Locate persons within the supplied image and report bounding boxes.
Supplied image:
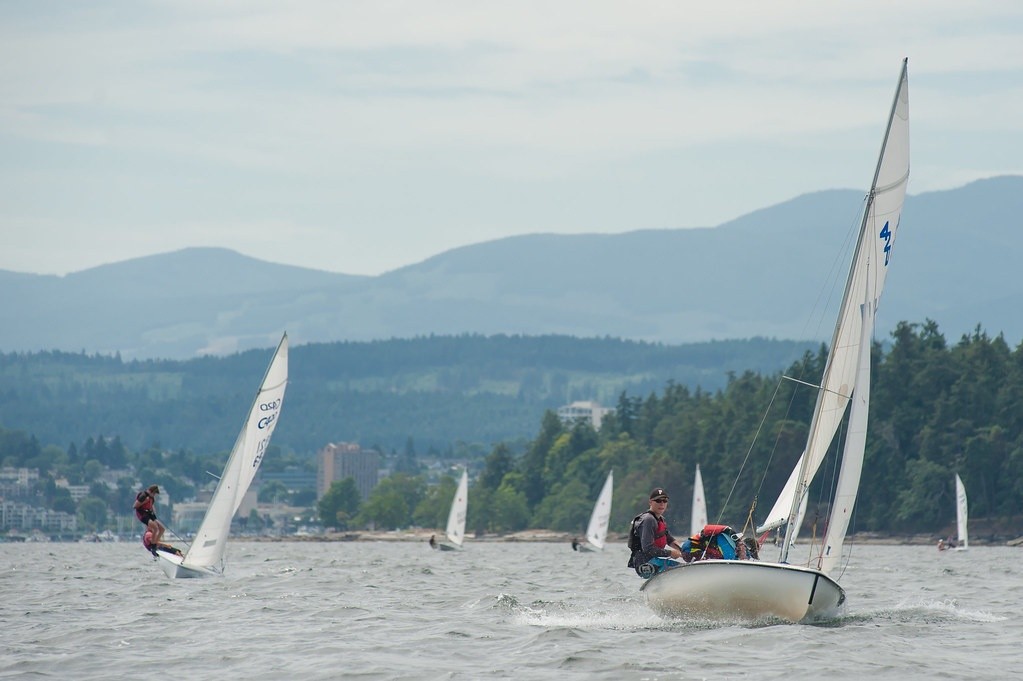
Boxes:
[430,535,434,545]
[132,485,165,558]
[573,539,579,550]
[682,526,760,563]
[143,525,171,552]
[938,539,947,551]
[627,488,682,576]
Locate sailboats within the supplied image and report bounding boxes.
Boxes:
[640,55,913,631]
[946,473,971,553]
[429,465,469,553]
[571,468,615,555]
[154,328,288,581]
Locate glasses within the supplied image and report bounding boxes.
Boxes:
[652,499,668,503]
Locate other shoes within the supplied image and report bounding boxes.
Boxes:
[152,543,159,557]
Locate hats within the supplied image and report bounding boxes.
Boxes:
[650,488,669,500]
[744,538,761,560]
